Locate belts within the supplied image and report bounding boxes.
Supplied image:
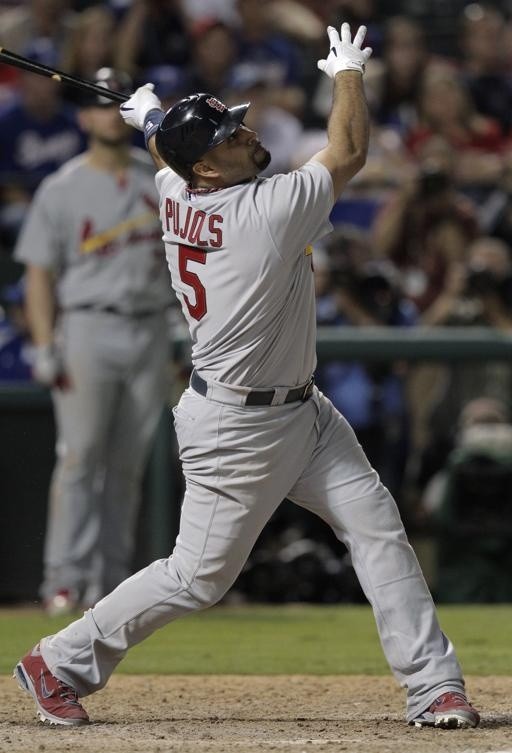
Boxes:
[188,371,317,407]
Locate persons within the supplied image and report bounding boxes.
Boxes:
[12,22,483,729]
[0,0,511,623]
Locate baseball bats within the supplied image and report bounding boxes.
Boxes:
[0,47,129,107]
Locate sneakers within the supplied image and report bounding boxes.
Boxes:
[412,691,480,729]
[11,641,90,726]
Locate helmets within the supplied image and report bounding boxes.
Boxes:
[155,92,251,181]
[73,67,133,107]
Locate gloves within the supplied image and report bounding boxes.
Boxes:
[31,344,63,386]
[316,21,373,79]
[119,81,164,131]
